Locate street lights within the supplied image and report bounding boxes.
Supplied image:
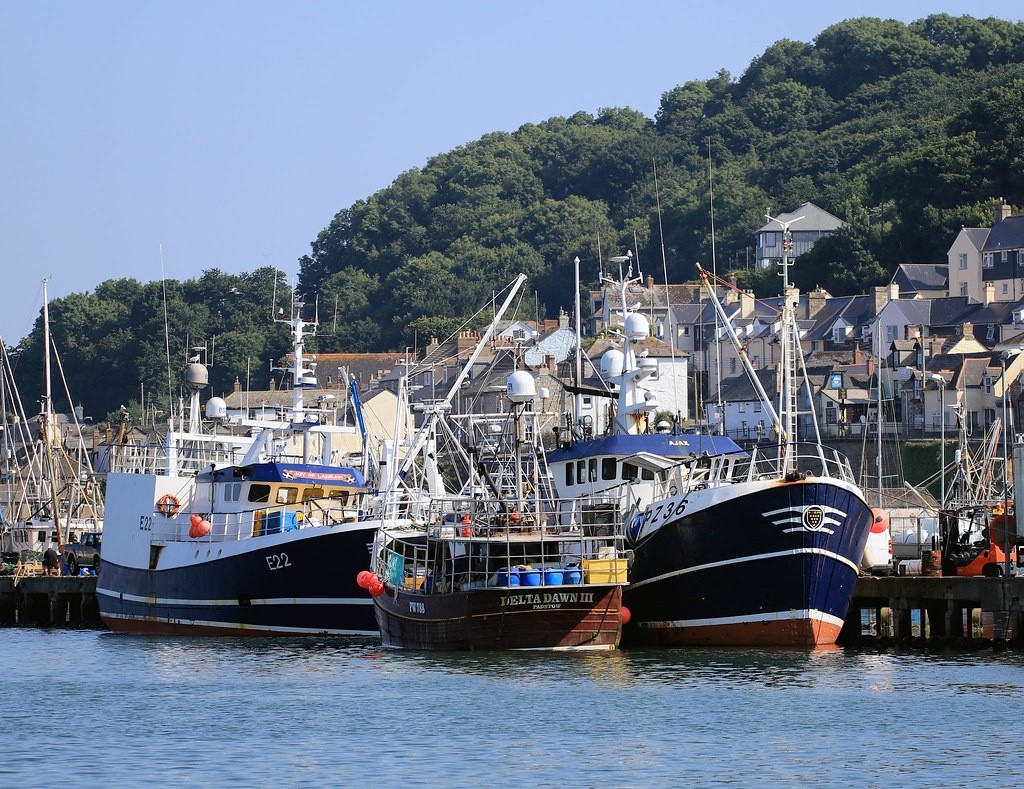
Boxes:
[926,374,946,511]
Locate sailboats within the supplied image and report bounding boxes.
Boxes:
[0,275,106,599]
[846,308,1014,555]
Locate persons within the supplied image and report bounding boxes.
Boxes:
[756,421,762,442]
[838,416,847,438]
[859,414,873,433]
[41,548,58,576]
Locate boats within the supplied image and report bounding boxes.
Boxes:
[368,272,632,647]
[95,244,387,639]
[538,230,754,526]
[610,135,874,648]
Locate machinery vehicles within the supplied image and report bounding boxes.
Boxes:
[939,504,1017,577]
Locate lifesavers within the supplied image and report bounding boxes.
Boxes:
[158,494,180,516]
